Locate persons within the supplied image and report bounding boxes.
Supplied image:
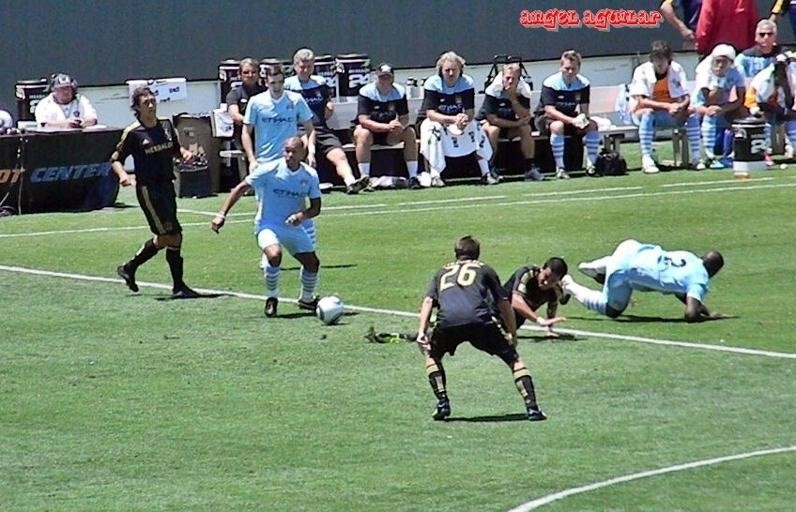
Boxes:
[212,137,321,316]
[35,74,97,128]
[111,87,196,297]
[416,235,545,421]
[557,239,723,321]
[227,0,795,193]
[497,257,569,337]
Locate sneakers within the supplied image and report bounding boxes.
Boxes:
[560,263,605,305]
[173,285,198,297]
[528,409,547,421]
[409,175,445,189]
[347,176,373,194]
[586,164,596,176]
[432,401,450,420]
[525,168,545,181]
[481,166,504,185]
[298,295,319,310]
[642,156,659,173]
[264,297,278,316]
[118,263,138,292]
[688,157,724,170]
[556,166,569,178]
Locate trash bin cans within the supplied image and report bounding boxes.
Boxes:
[731,118,767,161]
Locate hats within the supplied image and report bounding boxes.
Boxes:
[377,63,394,77]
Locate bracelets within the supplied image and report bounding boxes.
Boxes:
[537,317,545,326]
[216,214,228,219]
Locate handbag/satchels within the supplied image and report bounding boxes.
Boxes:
[597,148,627,176]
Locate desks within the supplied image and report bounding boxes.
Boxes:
[0,123,125,217]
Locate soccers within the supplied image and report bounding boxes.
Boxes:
[317,297,344,325]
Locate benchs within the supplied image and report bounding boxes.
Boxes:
[209,76,755,176]
